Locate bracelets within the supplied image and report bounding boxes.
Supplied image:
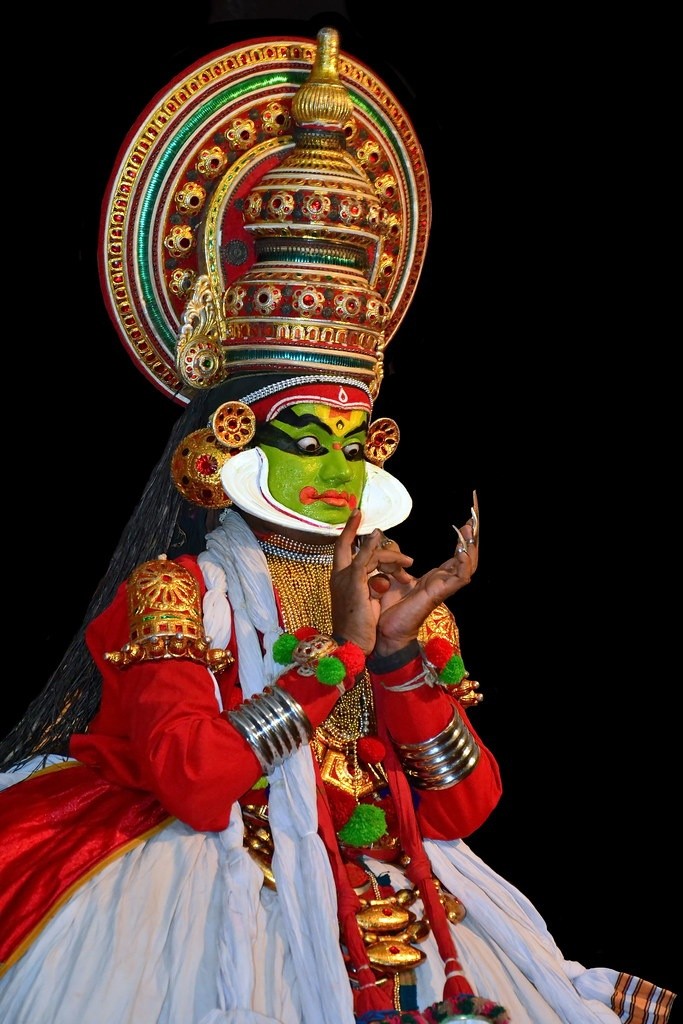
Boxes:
[380,640,484,709]
[272,626,367,694]
[391,704,481,791]
[225,684,312,776]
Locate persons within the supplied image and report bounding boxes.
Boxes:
[0,28,683,1024]
